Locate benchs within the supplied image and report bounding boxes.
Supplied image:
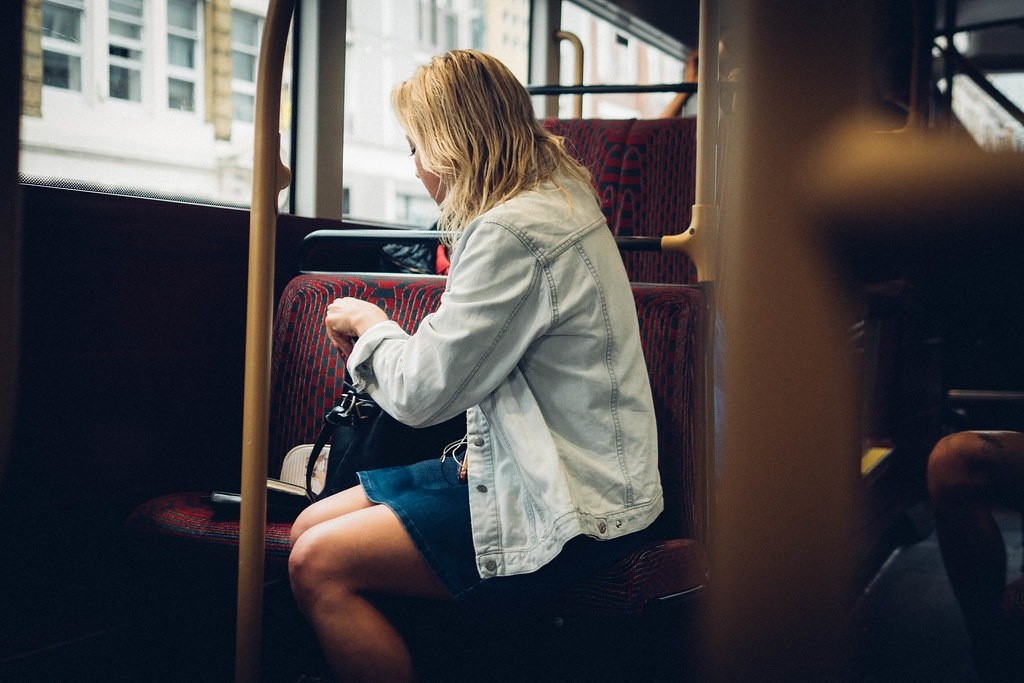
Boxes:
[432,118,700,288]
[127,267,711,626]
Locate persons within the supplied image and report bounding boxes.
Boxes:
[288,49,664,683]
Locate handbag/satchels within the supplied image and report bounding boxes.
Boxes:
[305,366,466,507]
